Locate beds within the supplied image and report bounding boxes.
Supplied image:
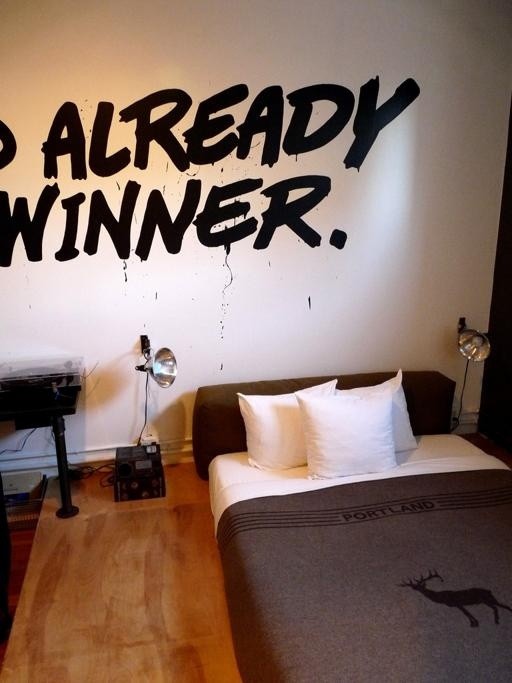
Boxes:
[179,367,512,683]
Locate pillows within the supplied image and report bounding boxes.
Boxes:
[294,387,398,480]
[234,377,339,470]
[335,368,419,453]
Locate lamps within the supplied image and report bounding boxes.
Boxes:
[135,334,178,447]
[449,315,491,434]
